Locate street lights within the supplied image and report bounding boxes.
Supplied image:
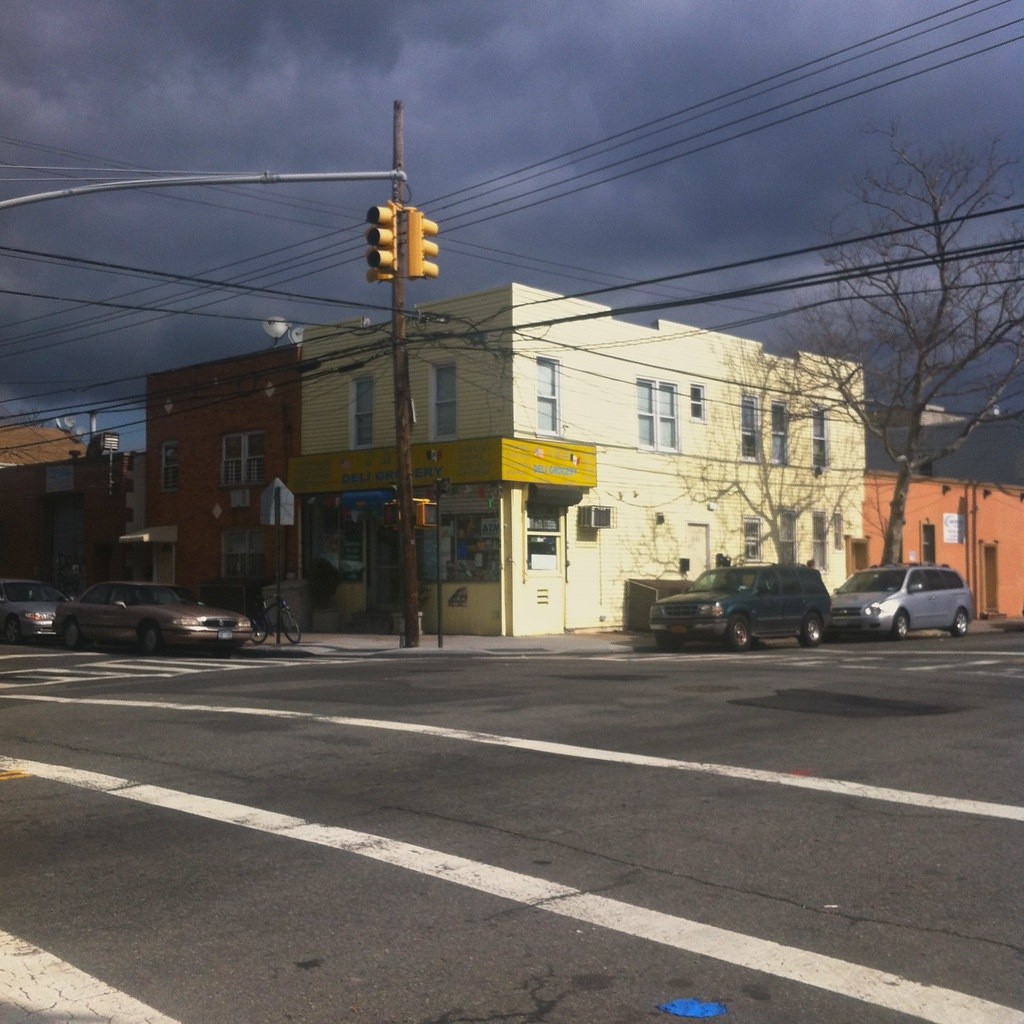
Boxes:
[432,477,452,648]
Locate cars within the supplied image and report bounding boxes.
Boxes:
[51,581,252,655]
[0,579,74,644]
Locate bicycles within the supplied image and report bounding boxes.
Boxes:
[249,591,301,645]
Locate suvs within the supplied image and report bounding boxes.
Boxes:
[647,561,832,653]
[824,563,977,641]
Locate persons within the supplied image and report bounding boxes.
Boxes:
[805,560,815,571]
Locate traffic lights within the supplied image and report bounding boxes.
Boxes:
[366,206,394,269]
